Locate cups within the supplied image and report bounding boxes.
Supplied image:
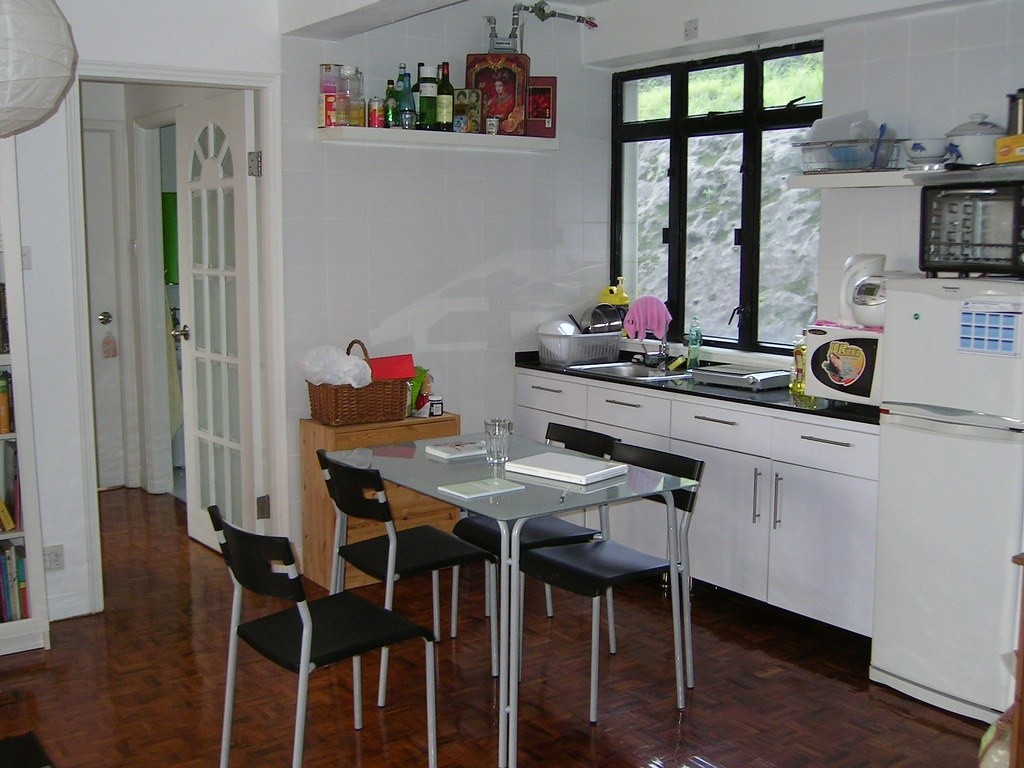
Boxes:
[484,418,509,464]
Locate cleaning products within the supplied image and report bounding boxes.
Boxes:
[599,276,631,337]
[686,315,702,373]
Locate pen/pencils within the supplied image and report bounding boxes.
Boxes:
[559,488,570,501]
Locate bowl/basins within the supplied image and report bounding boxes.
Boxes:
[539,320,582,361]
[944,113,1006,165]
[902,137,952,157]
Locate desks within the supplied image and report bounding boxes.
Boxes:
[322,431,700,768]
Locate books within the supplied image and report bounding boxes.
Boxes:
[438,477,525,499]
[426,440,487,459]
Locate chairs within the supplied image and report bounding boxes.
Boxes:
[207,504,438,768]
[313,448,498,709]
[450,421,622,640]
[515,440,705,725]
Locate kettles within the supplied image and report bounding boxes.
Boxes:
[837,253,887,327]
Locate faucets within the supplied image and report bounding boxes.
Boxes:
[627,318,669,371]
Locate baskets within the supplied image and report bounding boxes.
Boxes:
[305,339,408,426]
[536,331,620,368]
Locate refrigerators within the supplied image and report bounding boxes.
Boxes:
[867,275,1024,723]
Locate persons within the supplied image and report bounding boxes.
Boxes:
[456,90,480,103]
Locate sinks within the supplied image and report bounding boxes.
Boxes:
[566,361,693,380]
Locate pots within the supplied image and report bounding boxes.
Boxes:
[580,301,623,338]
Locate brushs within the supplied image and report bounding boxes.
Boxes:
[672,379,683,386]
[667,355,687,370]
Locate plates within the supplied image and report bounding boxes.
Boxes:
[907,157,951,165]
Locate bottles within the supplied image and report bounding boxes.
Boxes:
[428,395,443,417]
[416,393,434,412]
[335,65,362,126]
[385,62,454,131]
[485,116,500,135]
[1006,86,1024,137]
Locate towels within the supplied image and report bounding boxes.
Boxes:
[623,295,672,342]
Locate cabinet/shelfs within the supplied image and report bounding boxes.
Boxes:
[508,367,880,642]
[297,409,462,593]
[0,134,53,656]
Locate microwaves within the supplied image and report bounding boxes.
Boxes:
[917,182,1023,280]
